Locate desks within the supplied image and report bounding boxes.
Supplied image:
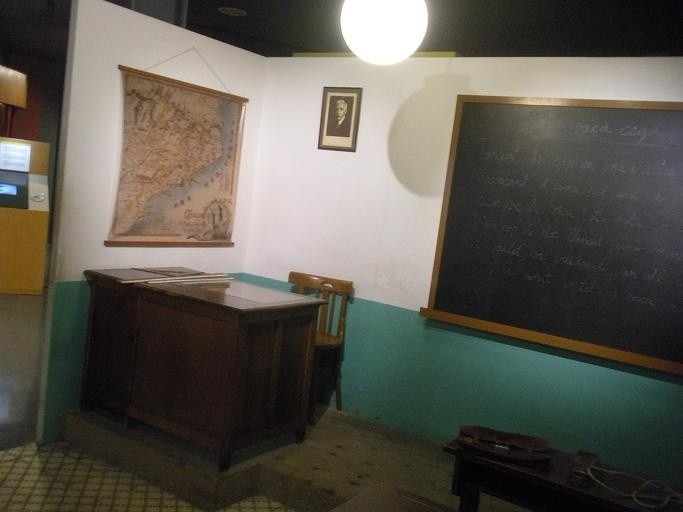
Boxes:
[443,419,683,512]
[79,264,330,476]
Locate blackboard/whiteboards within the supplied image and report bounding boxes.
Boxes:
[419,94,683,377]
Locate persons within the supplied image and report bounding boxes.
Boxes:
[325,99,349,137]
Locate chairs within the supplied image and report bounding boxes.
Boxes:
[284,268,354,426]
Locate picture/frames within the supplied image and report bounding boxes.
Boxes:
[317,85,364,153]
